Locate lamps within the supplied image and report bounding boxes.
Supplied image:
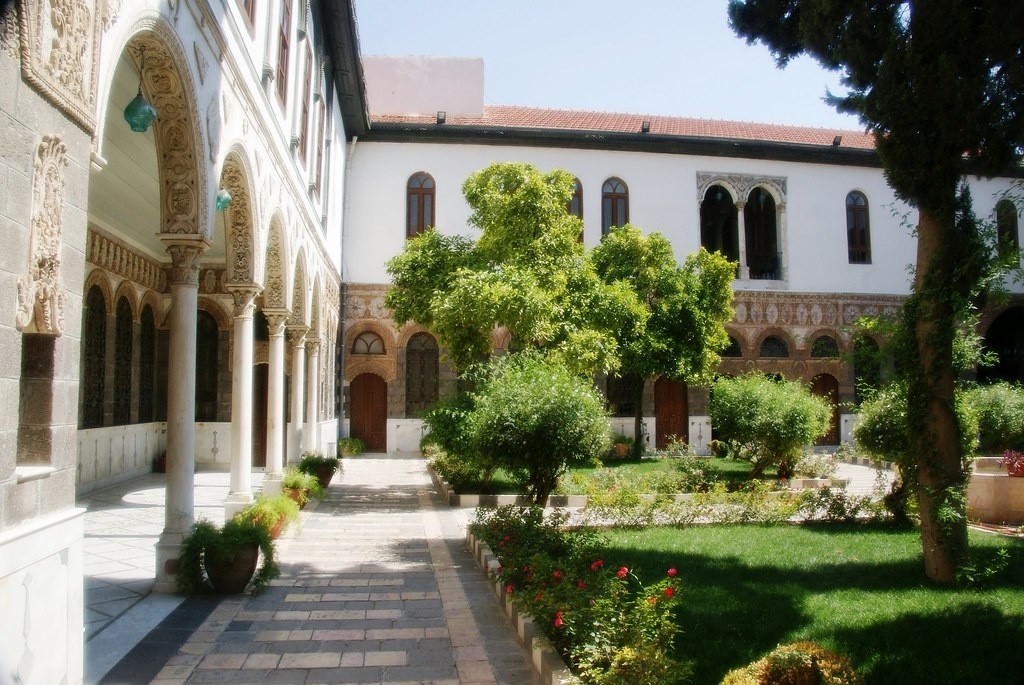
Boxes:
[124,45,156,131]
[437,111,446,123]
[833,136,842,145]
[216,161,231,211]
[642,121,650,131]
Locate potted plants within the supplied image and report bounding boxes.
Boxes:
[299,453,344,487]
[233,494,300,538]
[280,466,330,510]
[419,434,436,454]
[613,435,633,457]
[339,437,364,458]
[158,451,167,473]
[177,518,281,595]
